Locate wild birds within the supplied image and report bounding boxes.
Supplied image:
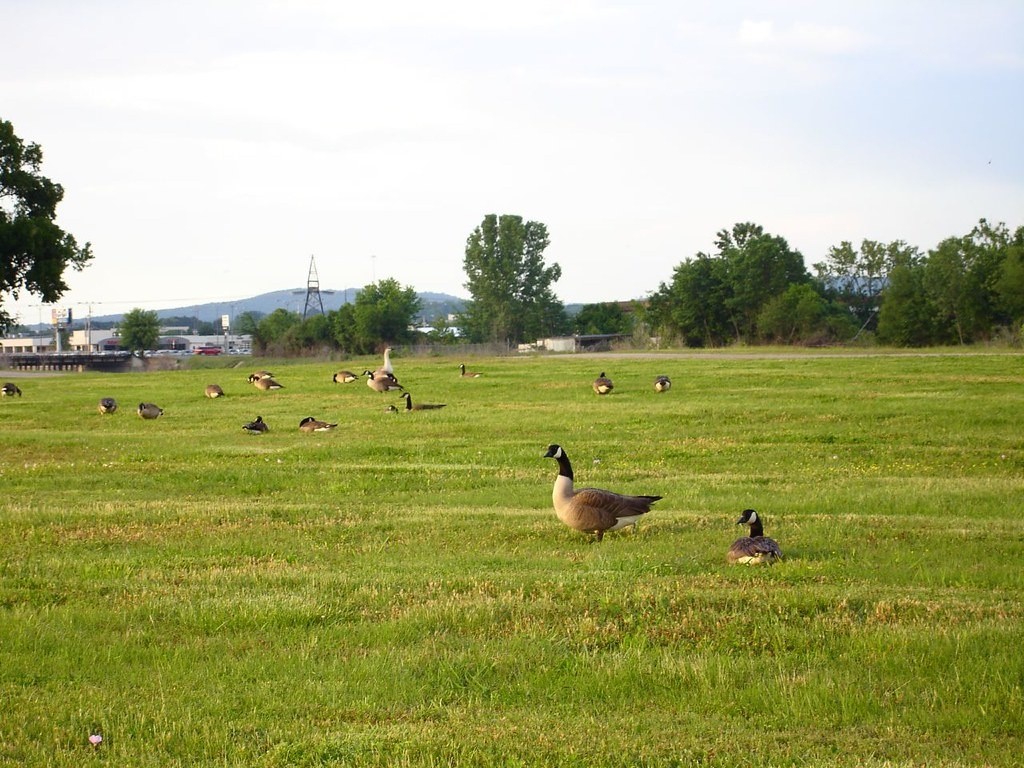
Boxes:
[333,346,404,393]
[138,403,164,420]
[2,383,22,398]
[98,398,117,415]
[727,509,784,563]
[544,444,663,543]
[205,384,225,399]
[593,372,613,394]
[299,416,338,432]
[458,364,482,378]
[241,416,268,434]
[384,392,446,413]
[655,375,671,390]
[248,370,286,390]
